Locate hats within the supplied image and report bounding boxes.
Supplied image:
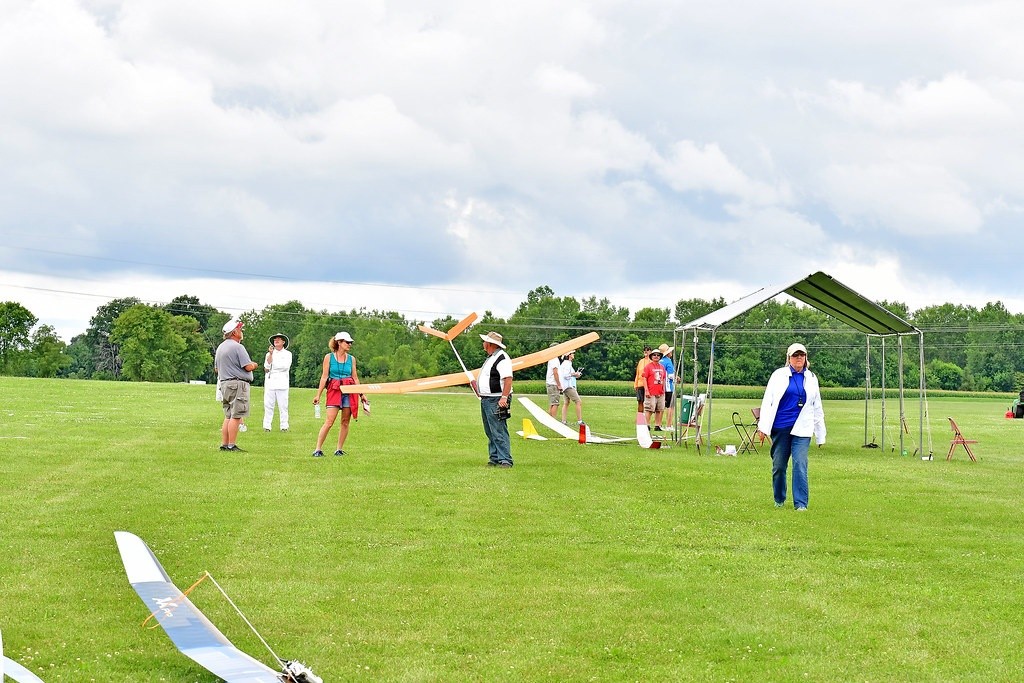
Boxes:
[561,350,576,360]
[649,348,663,360]
[788,343,807,356]
[479,332,506,350]
[643,346,651,350]
[658,344,674,357]
[268,333,290,350]
[334,332,355,342]
[222,320,243,334]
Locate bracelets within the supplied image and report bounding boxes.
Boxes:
[502,395,507,399]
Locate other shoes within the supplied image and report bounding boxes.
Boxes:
[334,450,348,456]
[561,420,586,426]
[220,446,227,450]
[281,429,287,432]
[797,507,807,511]
[226,445,247,452]
[774,501,784,510]
[313,450,324,457]
[487,462,496,466]
[265,429,270,432]
[501,463,510,468]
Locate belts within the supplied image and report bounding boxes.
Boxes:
[221,378,251,384]
[480,396,490,399]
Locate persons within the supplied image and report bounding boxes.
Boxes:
[312,332,368,459]
[633,343,680,432]
[545,343,562,419]
[262,332,293,432]
[756,343,826,510]
[558,349,586,425]
[213,318,259,453]
[478,331,514,469]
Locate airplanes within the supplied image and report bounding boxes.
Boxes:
[339,312,600,402]
[515,397,638,444]
[0,530,325,683]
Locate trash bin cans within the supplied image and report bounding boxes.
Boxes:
[682,395,701,424]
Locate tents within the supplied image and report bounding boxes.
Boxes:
[673,269,924,456]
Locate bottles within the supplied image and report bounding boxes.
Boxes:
[315,404,320,418]
[238,423,247,432]
[891,444,895,452]
[579,422,586,443]
[903,450,907,456]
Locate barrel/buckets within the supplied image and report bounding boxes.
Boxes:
[681,395,700,424]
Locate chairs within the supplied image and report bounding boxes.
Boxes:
[732,408,772,454]
[946,417,978,462]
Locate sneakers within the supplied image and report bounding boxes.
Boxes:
[647,417,680,432]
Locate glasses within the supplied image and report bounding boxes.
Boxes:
[341,341,352,345]
[643,349,650,351]
[792,352,805,357]
[570,352,575,354]
[651,354,660,356]
